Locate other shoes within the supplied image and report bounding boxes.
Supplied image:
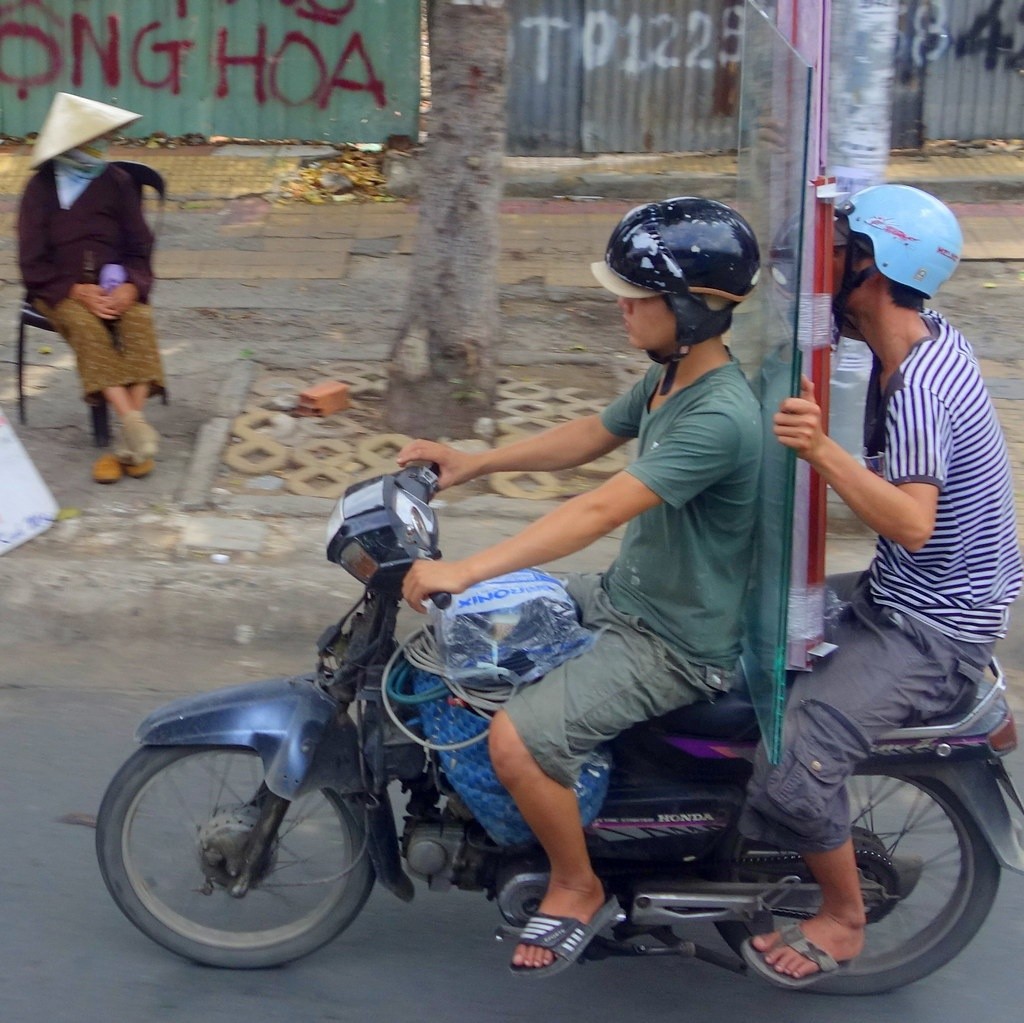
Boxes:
[91,450,122,482]
[121,455,155,477]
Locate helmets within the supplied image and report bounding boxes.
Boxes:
[833,185,962,298]
[604,198,762,302]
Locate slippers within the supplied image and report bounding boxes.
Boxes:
[507,892,621,980]
[740,921,856,988]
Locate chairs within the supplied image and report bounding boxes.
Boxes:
[16,160,166,449]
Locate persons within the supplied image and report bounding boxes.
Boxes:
[739,183,1024,990]
[395,196,764,981]
[17,92,170,467]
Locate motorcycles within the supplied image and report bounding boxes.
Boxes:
[94,456,1024,995]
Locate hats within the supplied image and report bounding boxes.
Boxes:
[26,93,141,169]
[588,259,732,311]
